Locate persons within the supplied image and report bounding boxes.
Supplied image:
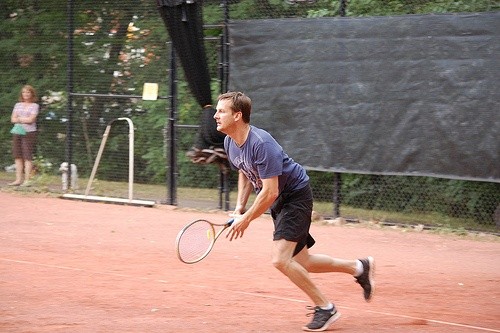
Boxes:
[9,86,39,187]
[213,91,372,331]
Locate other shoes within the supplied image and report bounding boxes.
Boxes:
[8,182,21,186]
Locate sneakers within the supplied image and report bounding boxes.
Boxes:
[354,256,375,303]
[303,302,340,331]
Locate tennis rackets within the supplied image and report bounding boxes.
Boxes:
[176,217,234,264]
